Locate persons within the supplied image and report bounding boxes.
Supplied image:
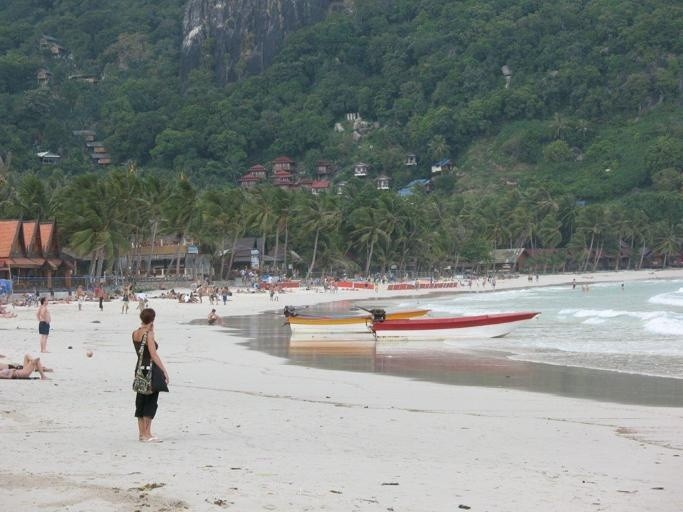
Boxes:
[0,264,589,329]
[132,309,169,443]
[0,354,52,372]
[0,352,50,380]
[36,297,51,353]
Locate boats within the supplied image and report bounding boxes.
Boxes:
[286,331,382,353]
[356,302,540,339]
[372,338,518,374]
[280,305,433,335]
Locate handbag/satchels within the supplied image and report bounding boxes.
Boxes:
[132,370,153,395]
